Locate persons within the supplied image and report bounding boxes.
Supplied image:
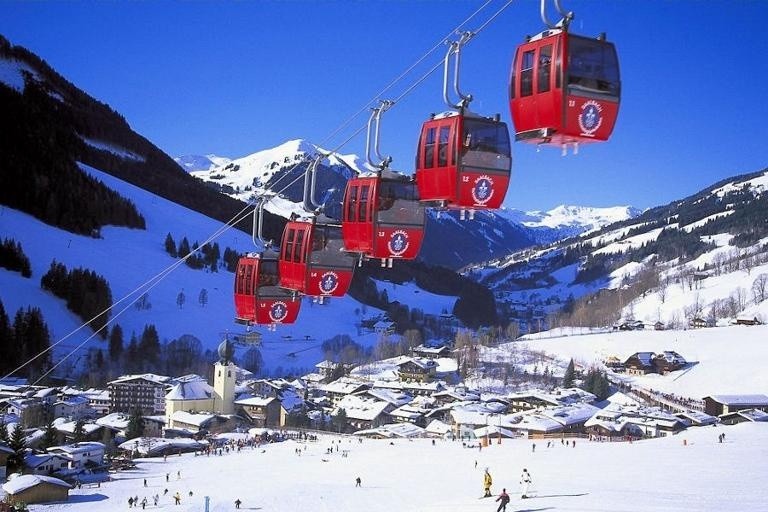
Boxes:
[532,438,575,452]
[628,436,632,444]
[722,433,725,438]
[474,459,478,468]
[194,428,317,456]
[179,449,182,456]
[164,454,167,461]
[495,488,510,512]
[128,471,194,509]
[719,434,722,443]
[520,468,532,499]
[235,499,241,508]
[483,467,492,498]
[78,482,81,489]
[321,437,363,462]
[356,477,361,487]
